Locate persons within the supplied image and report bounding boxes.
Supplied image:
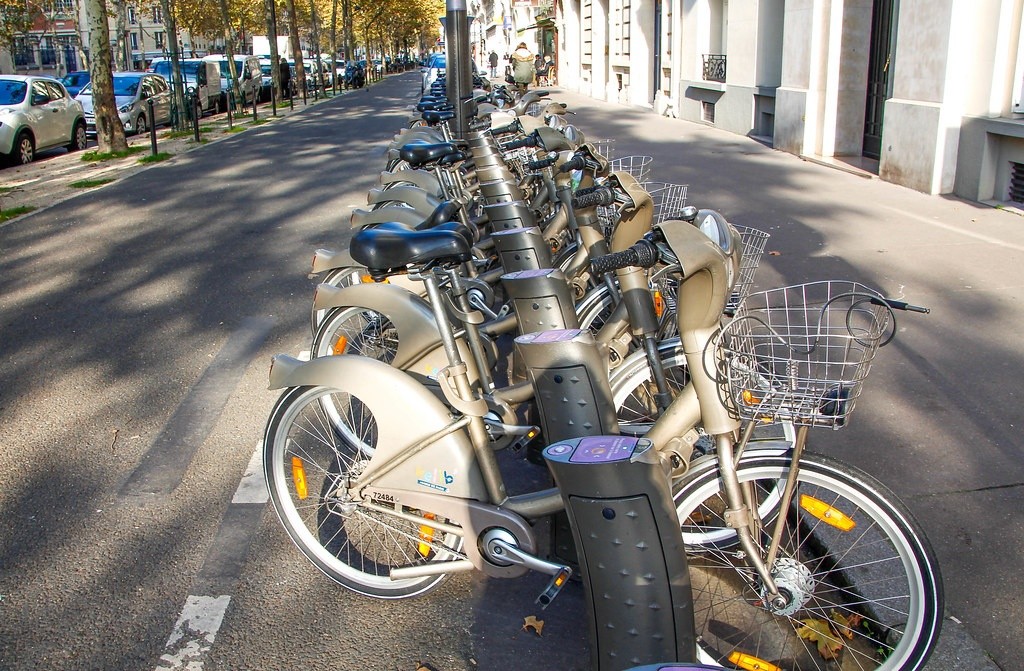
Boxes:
[395,56,400,63]
[279,58,290,100]
[487,49,498,78]
[345,62,363,84]
[510,42,554,99]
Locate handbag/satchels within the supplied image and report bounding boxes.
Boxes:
[505,74,516,84]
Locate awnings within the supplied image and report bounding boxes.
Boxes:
[517,15,555,32]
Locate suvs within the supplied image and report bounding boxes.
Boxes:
[203,53,264,109]
[153,58,222,120]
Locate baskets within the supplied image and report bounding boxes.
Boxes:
[607,155,654,185]
[652,222,771,310]
[637,181,690,225]
[590,138,616,162]
[713,276,890,430]
[522,102,548,117]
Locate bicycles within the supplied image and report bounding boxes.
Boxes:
[283,54,810,557]
[261,206,948,671]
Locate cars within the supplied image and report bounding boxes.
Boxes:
[0,72,88,165]
[39,69,90,99]
[258,57,280,87]
[420,54,489,94]
[74,70,176,139]
